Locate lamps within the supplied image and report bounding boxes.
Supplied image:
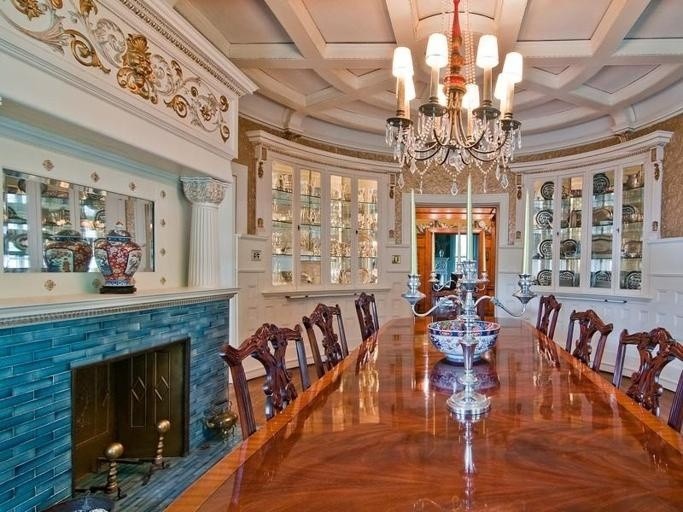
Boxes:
[377,1,525,196]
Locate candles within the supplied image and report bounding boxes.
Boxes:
[411,187,417,277]
[522,186,529,275]
[466,171,473,261]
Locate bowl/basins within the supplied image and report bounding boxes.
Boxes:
[426,318,501,365]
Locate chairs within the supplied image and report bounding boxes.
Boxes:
[219,322,312,441]
[536,294,562,338]
[564,308,613,370]
[354,291,380,341]
[612,327,682,432]
[302,303,350,378]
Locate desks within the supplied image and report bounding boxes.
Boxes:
[149,315,683,511]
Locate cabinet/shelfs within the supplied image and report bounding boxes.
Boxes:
[270,186,377,258]
[529,183,642,263]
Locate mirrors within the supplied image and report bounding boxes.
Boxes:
[0,168,155,277]
